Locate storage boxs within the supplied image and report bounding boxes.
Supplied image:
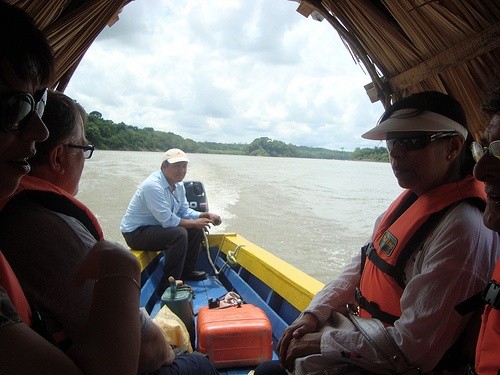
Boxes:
[197,302,273,368]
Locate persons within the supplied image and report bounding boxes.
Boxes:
[119,148,221,297]
[0,0,141,375]
[474,81,500,375]
[0,89,218,375]
[253,92,500,375]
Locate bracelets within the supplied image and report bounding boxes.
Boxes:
[95,273,142,295]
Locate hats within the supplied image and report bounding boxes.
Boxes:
[362,108,468,141]
[161,148,189,163]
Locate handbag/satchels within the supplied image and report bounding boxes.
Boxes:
[282,303,419,375]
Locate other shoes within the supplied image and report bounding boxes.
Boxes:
[183,270,207,281]
[157,284,196,297]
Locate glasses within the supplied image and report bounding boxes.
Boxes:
[0,92,46,133]
[385,131,461,152]
[63,142,95,159]
[469,140,500,162]
